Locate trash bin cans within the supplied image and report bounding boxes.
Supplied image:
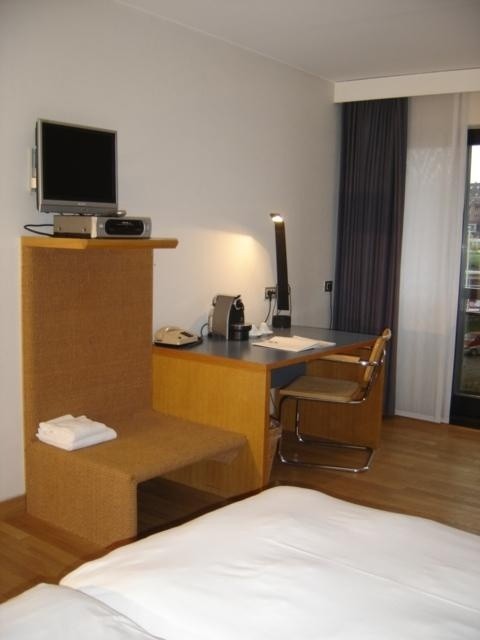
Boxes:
[265,415,283,482]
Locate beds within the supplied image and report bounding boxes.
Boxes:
[0,580,163,639]
[57,484,480,639]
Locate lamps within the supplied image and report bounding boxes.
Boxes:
[269,213,293,329]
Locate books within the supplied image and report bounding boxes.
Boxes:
[252,336,336,354]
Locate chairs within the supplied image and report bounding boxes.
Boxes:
[279,327,392,472]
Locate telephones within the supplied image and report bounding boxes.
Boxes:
[153,325,198,346]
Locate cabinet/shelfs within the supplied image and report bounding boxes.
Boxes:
[18,234,246,545]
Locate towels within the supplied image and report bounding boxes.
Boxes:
[37,409,106,441]
[36,417,117,454]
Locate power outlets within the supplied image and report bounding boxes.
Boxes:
[264,287,278,301]
[325,280,333,293]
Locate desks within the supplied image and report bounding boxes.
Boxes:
[154,323,384,497]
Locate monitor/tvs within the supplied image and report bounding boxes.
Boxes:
[31,118,118,214]
